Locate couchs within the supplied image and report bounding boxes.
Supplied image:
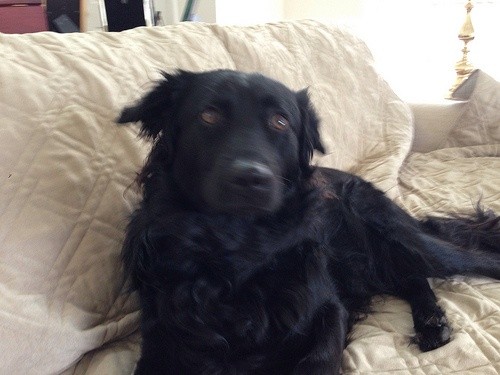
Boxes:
[2,19,500,375]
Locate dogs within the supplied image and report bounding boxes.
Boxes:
[114,67,500,374]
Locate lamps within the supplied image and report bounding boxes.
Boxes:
[451,0,474,91]
